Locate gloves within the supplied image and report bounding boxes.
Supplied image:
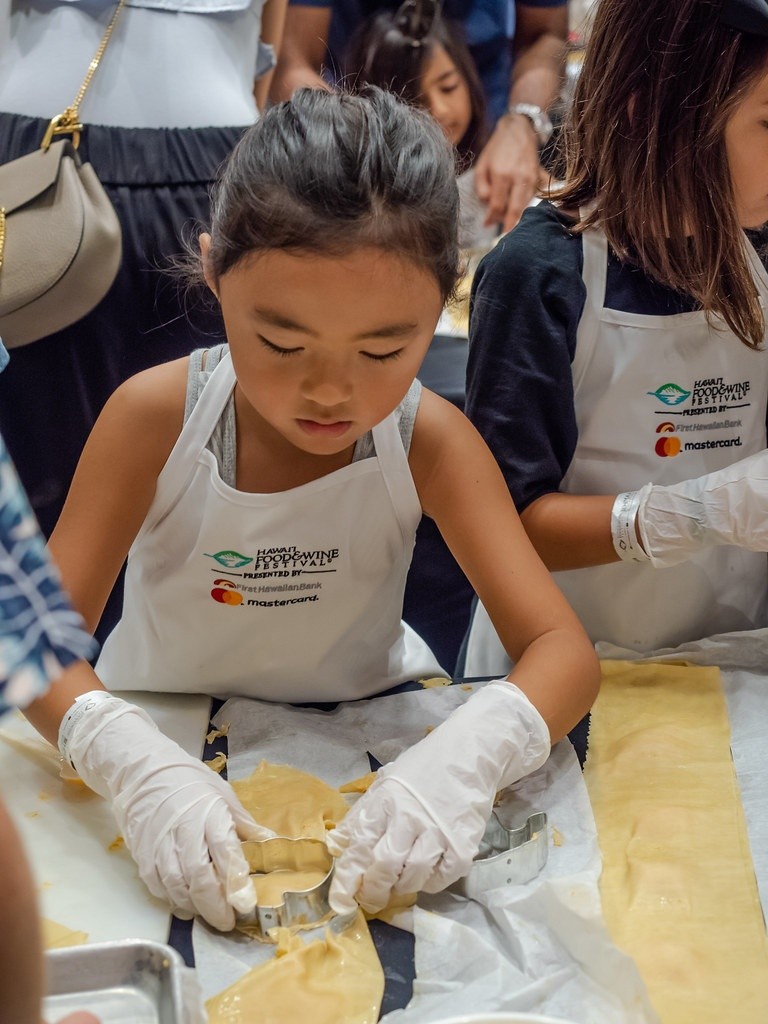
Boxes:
[327,683,550,915]
[638,448,768,568]
[70,697,277,932]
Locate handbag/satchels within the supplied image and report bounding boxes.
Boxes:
[1,139,124,350]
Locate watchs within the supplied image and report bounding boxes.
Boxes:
[507,102,555,147]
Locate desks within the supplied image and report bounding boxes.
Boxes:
[0,625,768,1023]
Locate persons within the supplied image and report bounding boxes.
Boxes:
[17,87,604,932]
[338,0,519,277]
[453,0,768,685]
[270,0,573,236]
[1,0,289,666]
